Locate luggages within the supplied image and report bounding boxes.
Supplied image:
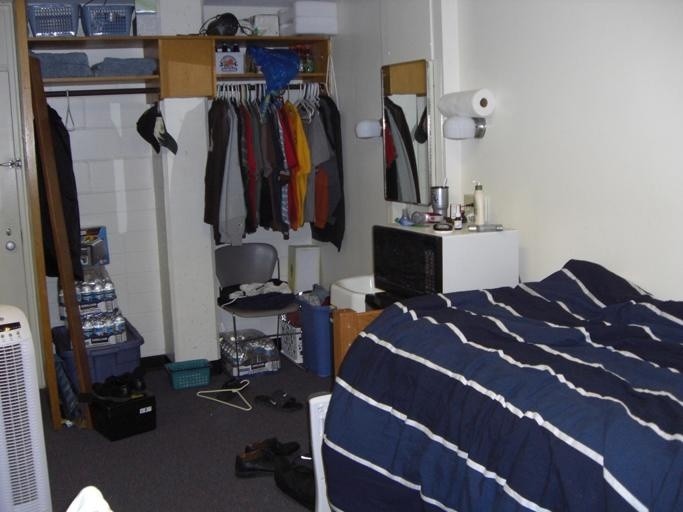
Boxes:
[219,328,281,378]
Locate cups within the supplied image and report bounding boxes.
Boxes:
[430,186,448,215]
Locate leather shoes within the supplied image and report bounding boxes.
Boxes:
[92,373,146,402]
[235,438,299,477]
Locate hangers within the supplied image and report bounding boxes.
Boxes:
[194,378,256,414]
[61,88,77,135]
[214,76,326,126]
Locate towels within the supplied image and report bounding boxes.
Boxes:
[37,50,158,78]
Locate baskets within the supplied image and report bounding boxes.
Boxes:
[164,359,214,391]
[81,3,135,36]
[25,1,80,36]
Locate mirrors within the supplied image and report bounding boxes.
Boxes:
[378,60,434,207]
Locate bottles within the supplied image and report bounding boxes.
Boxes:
[474,184,484,225]
[58,272,128,349]
[454,213,462,229]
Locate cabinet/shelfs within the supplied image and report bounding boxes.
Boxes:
[373,221,519,299]
[17,33,333,82]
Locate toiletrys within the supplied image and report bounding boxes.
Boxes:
[453,205,462,231]
[472,184,484,226]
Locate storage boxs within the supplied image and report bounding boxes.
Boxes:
[46,315,157,442]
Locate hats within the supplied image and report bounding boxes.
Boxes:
[137,104,178,155]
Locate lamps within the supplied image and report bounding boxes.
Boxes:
[354,118,382,140]
[444,116,487,140]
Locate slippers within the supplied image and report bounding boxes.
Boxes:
[218,378,247,401]
[255,391,302,412]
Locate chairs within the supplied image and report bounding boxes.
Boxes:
[214,242,310,379]
[307,283,682,512]
